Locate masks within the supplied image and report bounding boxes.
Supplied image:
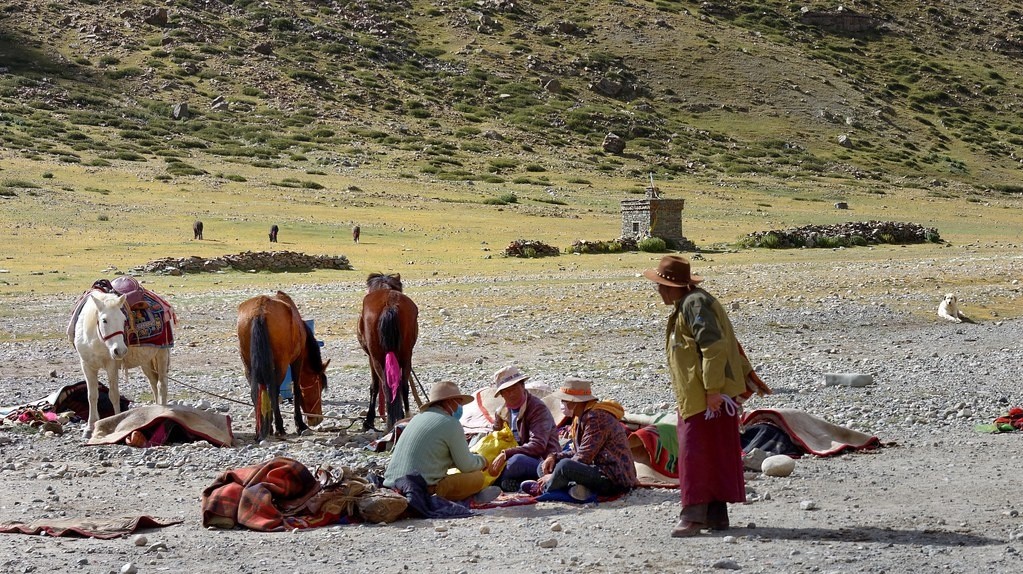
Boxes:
[559,402,577,417]
[446,398,463,420]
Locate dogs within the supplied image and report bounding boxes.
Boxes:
[937,293,970,324]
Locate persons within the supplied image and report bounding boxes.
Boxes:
[519,379,641,505]
[381,382,502,508]
[643,255,747,539]
[476,364,561,493]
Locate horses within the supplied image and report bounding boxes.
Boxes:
[356,272,419,432]
[269,225,279,242]
[235,291,332,441]
[352,227,360,243]
[73,278,171,439]
[194,222,203,241]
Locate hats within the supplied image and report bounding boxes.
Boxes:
[642,256,705,287]
[419,381,474,411]
[494,366,530,398]
[550,375,599,402]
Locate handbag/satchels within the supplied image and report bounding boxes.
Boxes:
[731,339,772,408]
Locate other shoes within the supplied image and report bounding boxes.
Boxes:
[700,523,729,530]
[473,486,501,504]
[501,480,521,491]
[670,520,702,537]
[569,484,592,500]
[521,480,541,496]
